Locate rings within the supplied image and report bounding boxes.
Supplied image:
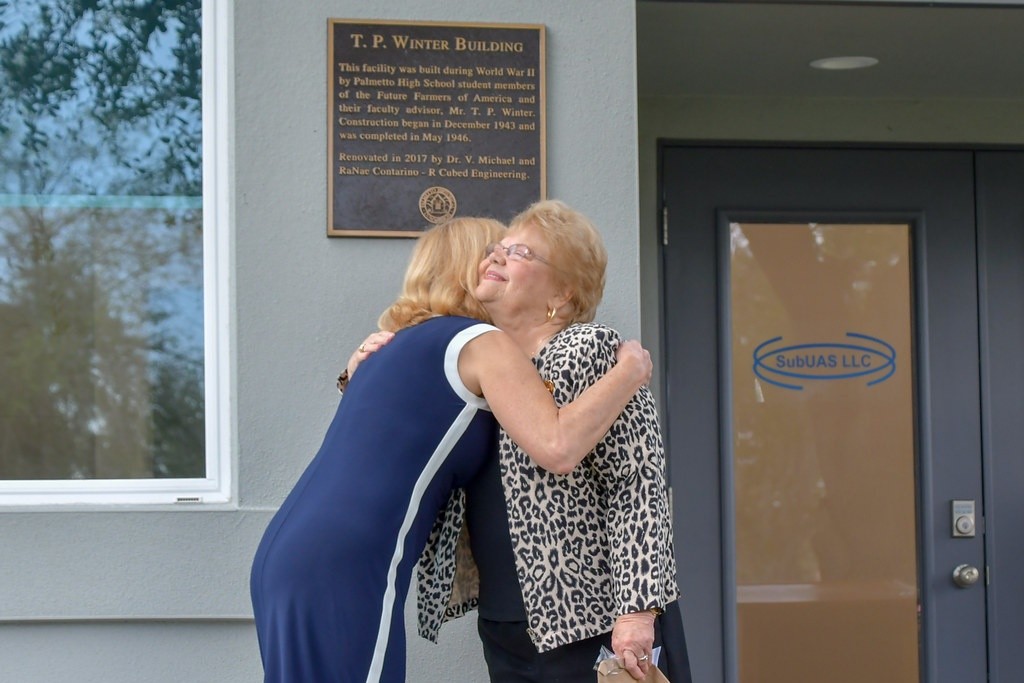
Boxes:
[638,656,648,661]
[359,344,366,352]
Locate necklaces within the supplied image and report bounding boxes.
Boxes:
[530,334,554,356]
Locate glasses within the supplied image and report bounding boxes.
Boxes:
[486,240,555,268]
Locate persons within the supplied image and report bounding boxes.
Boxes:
[251,216,653,683]
[337,200,693,683]
